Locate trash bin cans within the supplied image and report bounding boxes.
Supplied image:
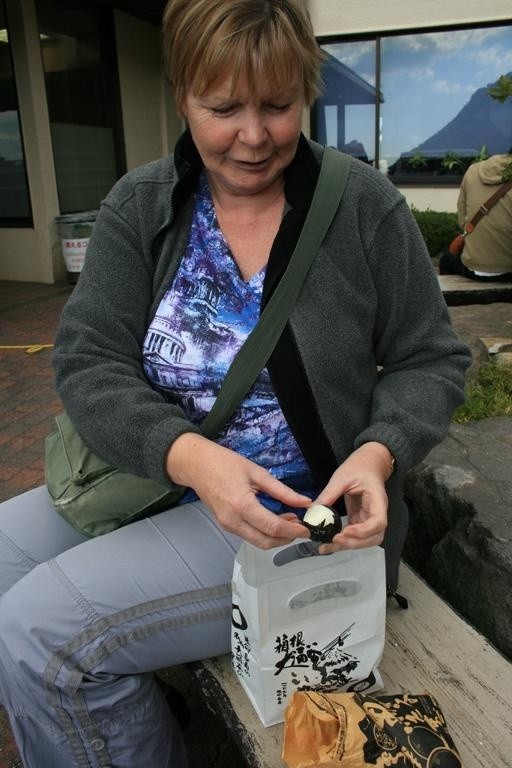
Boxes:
[54,209,100,284]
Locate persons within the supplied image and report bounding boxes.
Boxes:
[438,146,511,284]
[1,2,475,768]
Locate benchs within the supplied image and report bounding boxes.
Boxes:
[186,554,511,768]
[435,270,511,306]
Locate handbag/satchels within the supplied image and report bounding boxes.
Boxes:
[439,234,465,275]
[45,411,188,540]
[231,522,386,729]
